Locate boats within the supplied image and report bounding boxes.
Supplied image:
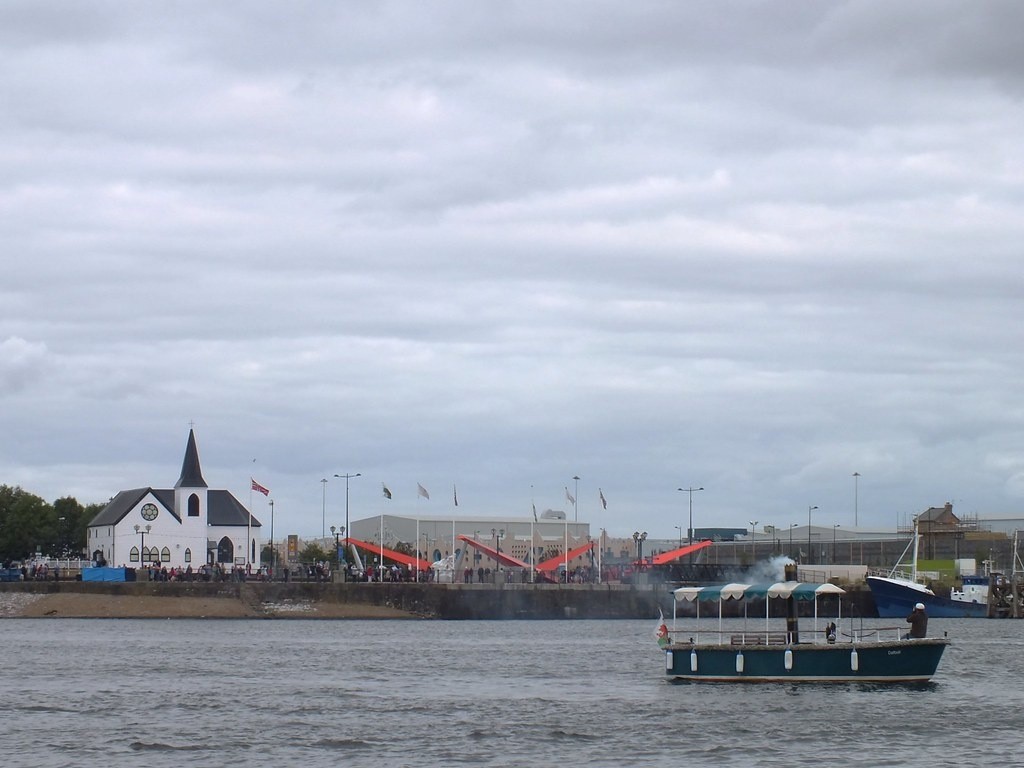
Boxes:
[656,578,953,685]
[865,512,990,619]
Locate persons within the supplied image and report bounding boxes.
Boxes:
[0,558,60,581]
[140,560,331,582]
[901,603,928,639]
[84,563,127,568]
[464,566,593,584]
[341,557,435,584]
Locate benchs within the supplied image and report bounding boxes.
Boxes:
[731,635,786,645]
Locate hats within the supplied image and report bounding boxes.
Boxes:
[915,603,925,610]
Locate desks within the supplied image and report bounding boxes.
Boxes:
[81,567,135,582]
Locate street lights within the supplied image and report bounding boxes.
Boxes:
[929,507,936,561]
[853,472,862,526]
[491,527,505,572]
[600,527,606,559]
[330,525,345,569]
[633,532,649,572]
[134,523,152,569]
[474,529,480,568]
[586,535,599,569]
[334,473,362,563]
[809,506,818,565]
[573,475,580,523]
[789,523,798,561]
[423,533,428,561]
[678,486,705,569]
[749,521,758,555]
[675,526,682,550]
[269,499,274,568]
[833,525,840,565]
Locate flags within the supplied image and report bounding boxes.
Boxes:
[251,479,269,496]
[383,483,391,499]
[600,489,606,509]
[418,483,429,500]
[566,488,574,506]
[454,484,458,506]
[532,503,537,522]
